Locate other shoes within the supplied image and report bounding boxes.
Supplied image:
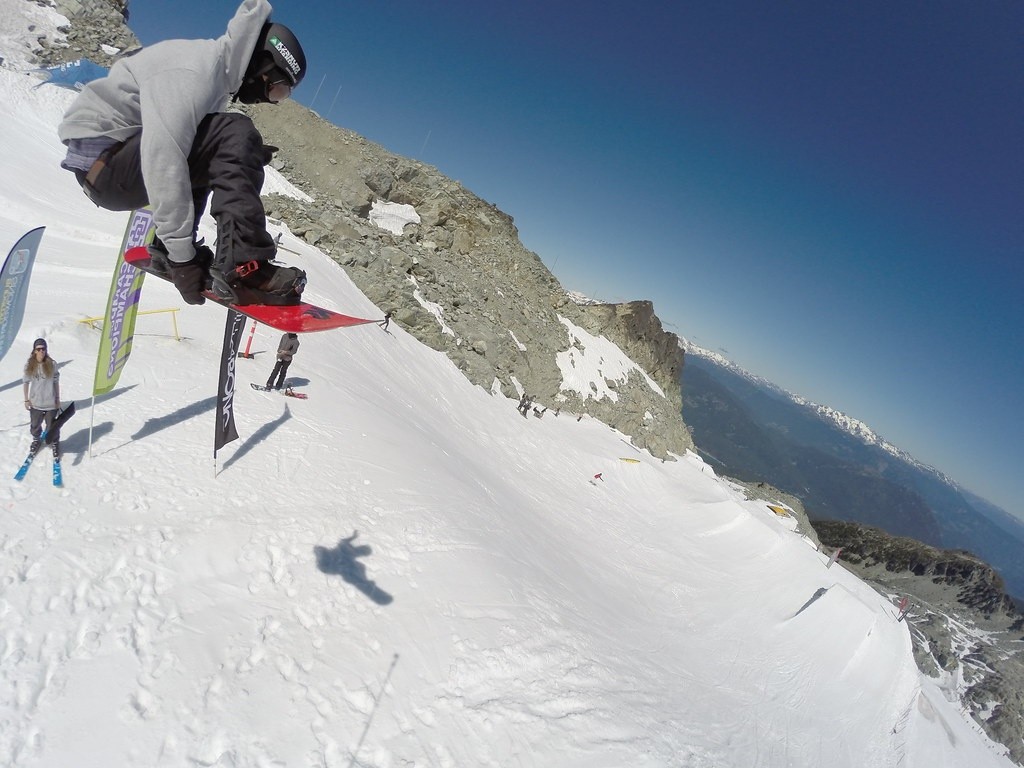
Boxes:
[275,382,283,390]
[266,384,273,391]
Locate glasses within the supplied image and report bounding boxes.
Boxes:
[35,347,46,351]
[213,260,306,300]
[263,57,291,102]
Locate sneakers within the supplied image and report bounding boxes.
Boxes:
[154,246,215,270]
[30,436,43,454]
[52,440,59,460]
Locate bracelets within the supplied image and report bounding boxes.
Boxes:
[56,396,60,398]
[24,400,29,402]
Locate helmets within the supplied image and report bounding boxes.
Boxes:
[256,24,306,87]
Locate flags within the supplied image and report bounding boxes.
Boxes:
[215,309,247,448]
[93,205,158,396]
[795,526,919,627]
[0,228,44,360]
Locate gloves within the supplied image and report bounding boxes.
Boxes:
[163,248,207,305]
[260,146,279,166]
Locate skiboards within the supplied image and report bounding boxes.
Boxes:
[376,322,396,339]
[14,419,63,486]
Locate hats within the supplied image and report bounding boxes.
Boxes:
[34,339,47,348]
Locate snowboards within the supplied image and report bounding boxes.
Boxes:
[125,246,385,335]
[250,383,307,400]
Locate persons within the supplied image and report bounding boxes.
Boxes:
[23,338,61,462]
[378,309,398,331]
[590,473,604,485]
[57,1,308,307]
[271,232,283,262]
[265,332,300,389]
[516,393,583,422]
